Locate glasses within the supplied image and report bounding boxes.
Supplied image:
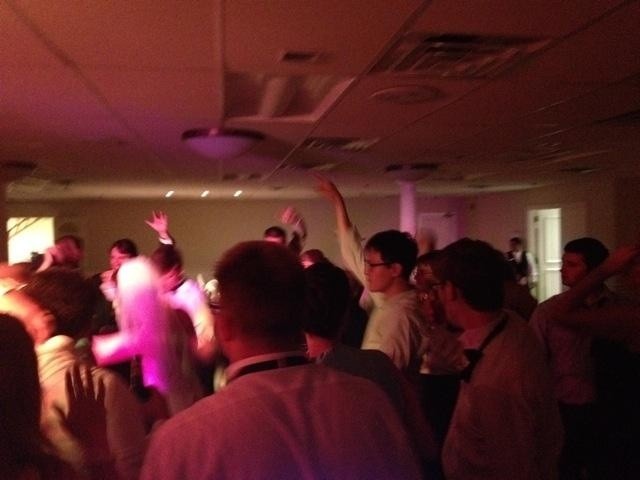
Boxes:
[363,259,391,269]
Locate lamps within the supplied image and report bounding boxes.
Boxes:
[180,126,268,162]
[385,162,437,182]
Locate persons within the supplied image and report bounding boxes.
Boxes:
[2,166,638,477]
